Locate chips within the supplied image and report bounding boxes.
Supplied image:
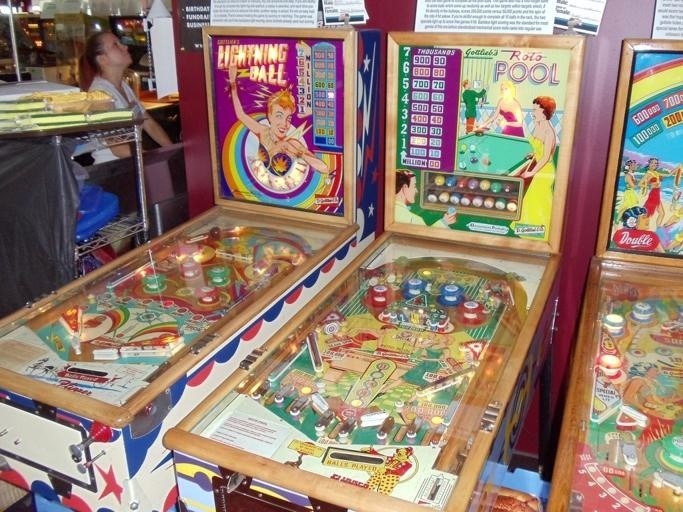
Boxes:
[43,90,113,112]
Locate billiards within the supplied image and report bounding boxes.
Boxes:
[434,176,515,193]
[427,192,517,212]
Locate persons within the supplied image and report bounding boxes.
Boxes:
[508,95,558,241]
[473,79,526,138]
[638,156,683,227]
[462,76,489,134]
[616,158,643,224]
[226,60,332,193]
[78,30,176,165]
[393,168,459,230]
